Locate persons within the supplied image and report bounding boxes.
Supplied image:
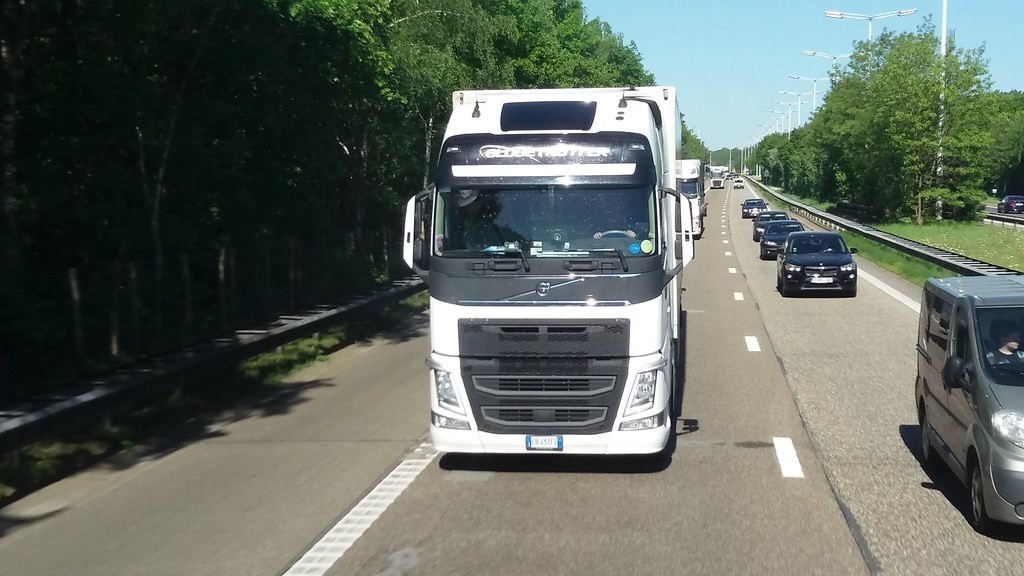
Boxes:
[985,319,1024,365]
[591,224,643,239]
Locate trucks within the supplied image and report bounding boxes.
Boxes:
[710,169,725,188]
[403,85,696,455]
[678,156,707,236]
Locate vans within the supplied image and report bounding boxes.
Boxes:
[912,272,1024,533]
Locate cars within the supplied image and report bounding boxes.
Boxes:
[751,211,797,242]
[775,231,858,298]
[997,195,1024,214]
[724,172,745,190]
[740,199,769,219]
[758,220,806,260]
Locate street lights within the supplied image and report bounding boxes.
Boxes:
[824,9,917,80]
[761,48,854,140]
